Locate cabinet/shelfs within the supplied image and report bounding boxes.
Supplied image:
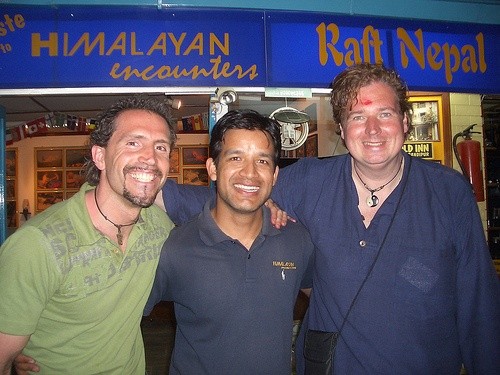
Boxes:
[5,97,320,238]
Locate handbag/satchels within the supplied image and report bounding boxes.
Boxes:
[304,328,336,374]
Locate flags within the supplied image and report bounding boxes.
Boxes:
[4,111,211,147]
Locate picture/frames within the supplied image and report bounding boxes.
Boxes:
[402,91,453,169]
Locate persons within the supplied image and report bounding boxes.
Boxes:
[153,59,500,375]
[0,95,298,375]
[15,108,318,375]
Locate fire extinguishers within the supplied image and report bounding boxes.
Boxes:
[453,124,486,202]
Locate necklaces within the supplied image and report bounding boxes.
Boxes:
[353,157,404,208]
[93,187,140,246]
